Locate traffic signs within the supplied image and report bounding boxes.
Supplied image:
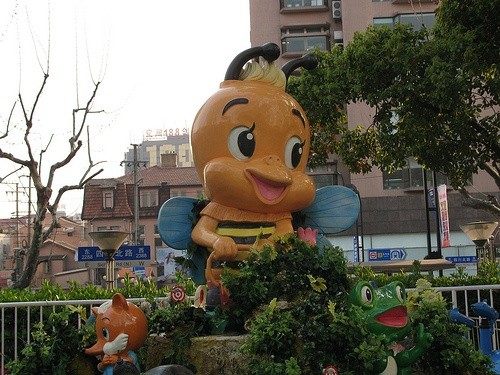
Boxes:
[77,245,151,268]
[445,256,477,267]
[369,248,407,261]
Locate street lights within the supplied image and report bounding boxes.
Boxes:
[88,231,131,291]
[457,220,499,277]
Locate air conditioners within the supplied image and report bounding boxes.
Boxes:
[332,0,341,19]
